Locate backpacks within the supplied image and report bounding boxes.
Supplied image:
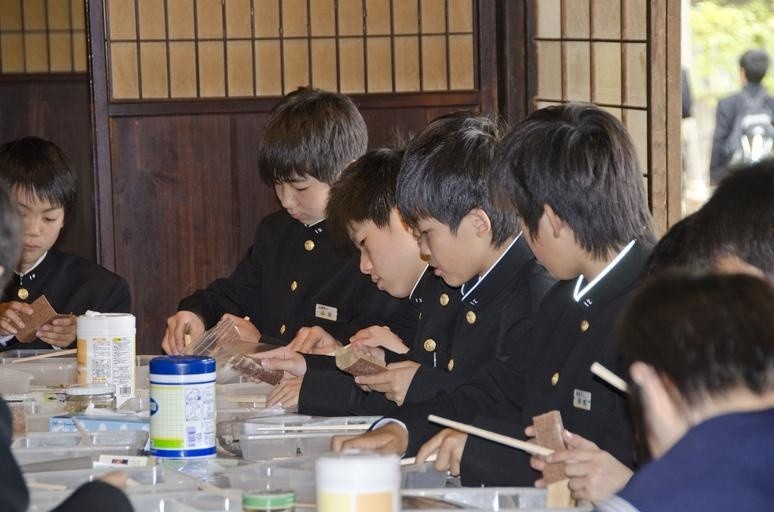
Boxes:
[727,92,770,170]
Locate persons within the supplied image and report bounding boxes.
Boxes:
[0,180,135,511]
[0,135,131,350]
[167,50,773,510]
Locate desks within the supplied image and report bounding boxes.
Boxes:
[1,355,471,512]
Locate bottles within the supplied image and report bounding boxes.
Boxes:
[64,382,117,415]
[146,352,219,458]
[237,492,295,511]
[75,310,136,405]
